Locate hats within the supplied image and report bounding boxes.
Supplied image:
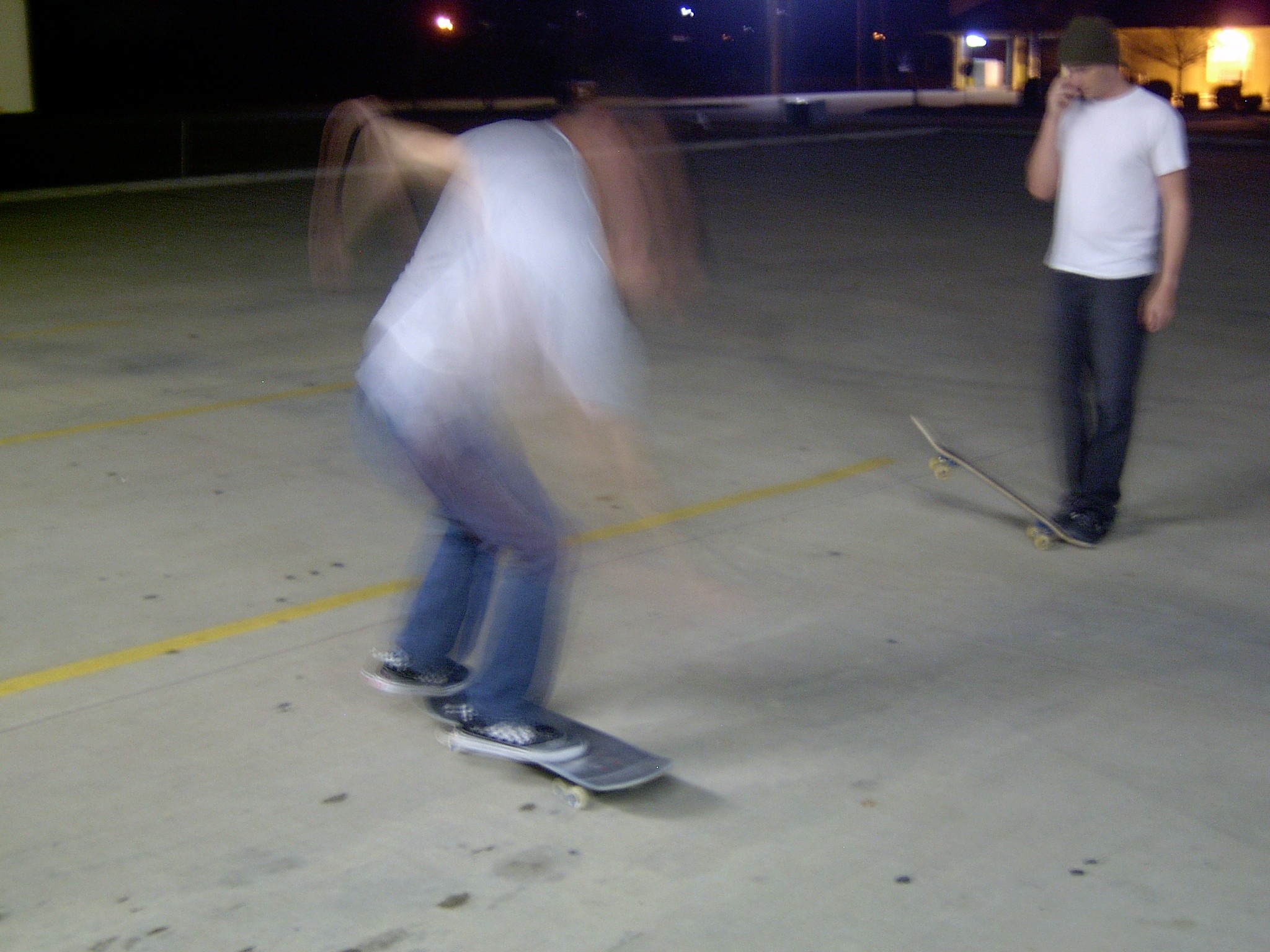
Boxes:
[1055,15,1119,65]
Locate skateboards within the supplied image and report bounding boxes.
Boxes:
[421,689,673,811]
[910,415,1096,550]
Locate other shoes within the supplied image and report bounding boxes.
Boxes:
[446,713,587,763]
[364,658,473,696]
[1051,498,1118,545]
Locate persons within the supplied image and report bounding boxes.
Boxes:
[1028,16,1191,546]
[323,96,681,761]
